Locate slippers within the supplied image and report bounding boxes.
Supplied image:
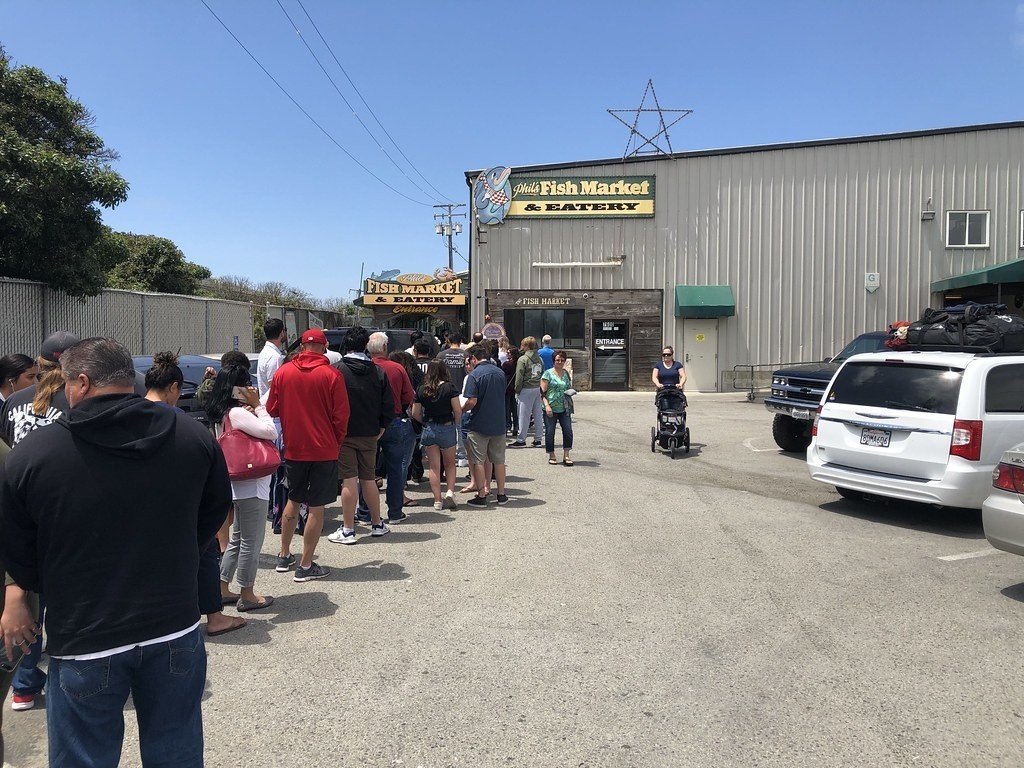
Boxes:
[564,459,573,466]
[549,458,558,465]
[208,617,246,636]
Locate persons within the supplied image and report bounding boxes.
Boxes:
[540,350,576,466]
[257,319,285,398]
[199,352,251,555]
[206,365,278,610]
[266,330,351,582]
[651,346,687,423]
[326,327,394,544]
[508,337,544,448]
[0,331,248,768]
[142,351,247,636]
[390,330,521,511]
[355,331,415,523]
[539,335,555,370]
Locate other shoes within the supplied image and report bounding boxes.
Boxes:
[237,596,273,612]
[222,594,240,604]
[531,441,542,448]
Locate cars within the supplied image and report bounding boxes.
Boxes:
[126,351,272,422]
[981,442,1024,557]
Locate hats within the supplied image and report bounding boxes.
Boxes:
[41,330,79,362]
[302,329,327,353]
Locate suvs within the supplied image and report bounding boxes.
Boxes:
[804,351,1024,516]
[761,328,890,450]
[288,326,442,379]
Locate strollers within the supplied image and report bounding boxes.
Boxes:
[651,384,690,459]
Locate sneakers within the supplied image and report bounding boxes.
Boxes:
[267,457,508,582]
[12,692,34,710]
[508,441,527,448]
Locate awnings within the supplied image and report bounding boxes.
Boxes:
[931,258,1024,291]
[675,285,735,318]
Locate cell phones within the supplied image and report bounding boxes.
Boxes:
[231,386,249,400]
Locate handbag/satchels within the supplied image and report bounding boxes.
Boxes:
[217,407,281,481]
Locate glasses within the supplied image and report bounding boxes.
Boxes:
[662,353,672,357]
[507,352,511,355]
[245,380,253,386]
